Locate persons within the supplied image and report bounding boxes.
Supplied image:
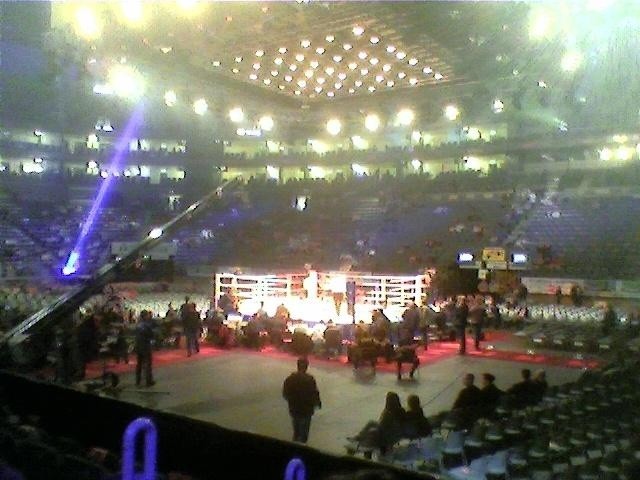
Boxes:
[135,308,156,385]
[0,409,190,477]
[1,135,638,270]
[282,357,322,442]
[341,281,639,455]
[78,292,341,365]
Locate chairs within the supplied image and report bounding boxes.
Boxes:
[0,407,168,480]
[344,363,640,480]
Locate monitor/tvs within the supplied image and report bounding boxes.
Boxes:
[512,252,529,265]
[457,248,475,264]
